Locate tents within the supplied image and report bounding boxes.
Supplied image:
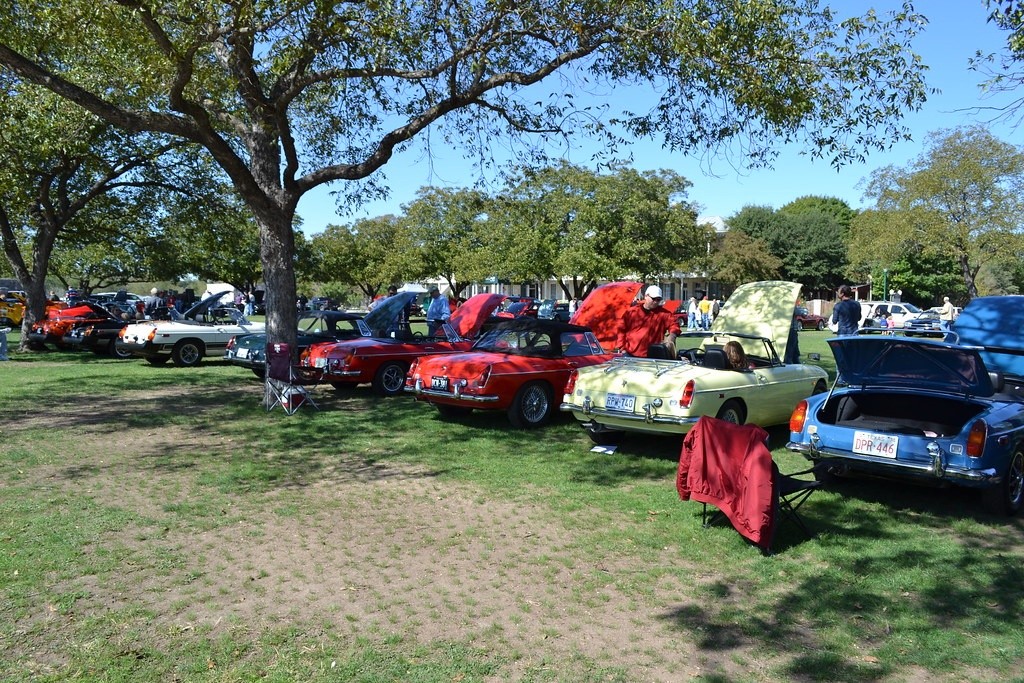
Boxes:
[396,282,428,295]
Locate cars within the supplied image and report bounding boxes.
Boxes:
[660,299,689,327]
[788,295,1024,517]
[0,275,583,368]
[794,306,828,333]
[903,307,963,338]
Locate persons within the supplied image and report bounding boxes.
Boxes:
[569,297,582,320]
[323,299,332,311]
[886,316,895,336]
[831,285,861,386]
[66,284,78,308]
[299,293,308,311]
[616,284,682,358]
[409,302,420,316]
[145,287,167,319]
[201,290,218,322]
[235,289,256,317]
[880,314,888,335]
[49,291,61,301]
[367,285,404,337]
[939,296,953,340]
[686,296,719,331]
[426,286,450,336]
[722,340,755,370]
[503,287,506,293]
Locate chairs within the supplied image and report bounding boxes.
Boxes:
[121,313,144,320]
[701,349,733,370]
[647,344,673,359]
[178,314,189,321]
[265,343,324,416]
[675,415,841,555]
[135,313,145,320]
[196,314,207,323]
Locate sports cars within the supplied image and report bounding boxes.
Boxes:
[403,281,689,430]
[223,310,371,386]
[299,291,512,397]
[558,280,828,453]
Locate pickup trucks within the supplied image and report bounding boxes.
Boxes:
[857,301,930,334]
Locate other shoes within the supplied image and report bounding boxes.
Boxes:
[687,327,705,332]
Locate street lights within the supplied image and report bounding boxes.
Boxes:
[882,266,888,301]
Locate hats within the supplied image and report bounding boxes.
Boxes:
[151,287,158,294]
[645,285,663,301]
[944,296,949,301]
[428,285,438,293]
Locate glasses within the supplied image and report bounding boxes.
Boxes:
[389,292,396,295]
[648,294,661,301]
